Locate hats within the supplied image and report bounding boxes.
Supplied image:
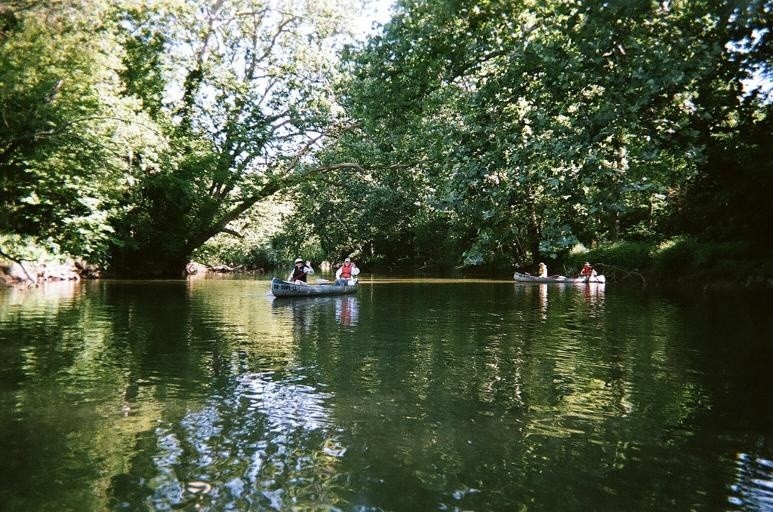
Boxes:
[295,258,303,263]
[345,258,350,262]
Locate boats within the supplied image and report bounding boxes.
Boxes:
[271,277,361,297]
[554,274,606,283]
[513,272,566,283]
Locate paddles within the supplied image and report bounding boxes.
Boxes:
[265,269,309,296]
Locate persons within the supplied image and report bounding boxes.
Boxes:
[335,258,360,285]
[537,262,548,278]
[580,261,592,279]
[287,258,314,285]
